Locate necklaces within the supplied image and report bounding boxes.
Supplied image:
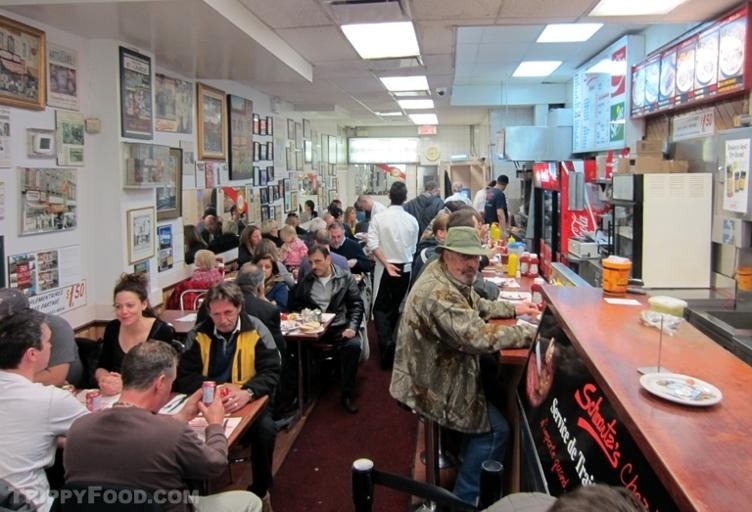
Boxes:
[112,400,158,415]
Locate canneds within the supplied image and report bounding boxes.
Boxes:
[201,380,216,405]
[217,385,228,405]
[85,389,101,411]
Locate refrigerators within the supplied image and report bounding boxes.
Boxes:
[612,173,714,289]
[533,160,612,283]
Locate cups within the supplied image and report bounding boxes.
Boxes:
[301,309,312,323]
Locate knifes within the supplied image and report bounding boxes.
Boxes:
[167,395,190,413]
[223,418,229,432]
[285,329,296,335]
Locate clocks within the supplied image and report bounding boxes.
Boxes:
[424,146,440,161]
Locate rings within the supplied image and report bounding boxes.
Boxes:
[234,402,239,407]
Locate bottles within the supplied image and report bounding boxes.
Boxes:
[529,278,544,309]
[505,251,518,277]
[500,248,510,272]
[496,239,505,253]
[508,234,515,243]
[490,222,500,240]
[518,251,530,276]
[527,253,539,279]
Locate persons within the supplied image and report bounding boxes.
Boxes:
[1,287,85,388]
[166,173,511,421]
[61,338,264,511]
[388,225,543,508]
[484,482,648,511]
[171,279,284,510]
[0,309,94,511]
[88,269,177,393]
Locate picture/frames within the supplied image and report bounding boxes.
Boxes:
[0,14,337,265]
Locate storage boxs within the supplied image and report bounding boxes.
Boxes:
[612,140,689,172]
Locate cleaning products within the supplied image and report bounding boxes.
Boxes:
[490,221,501,239]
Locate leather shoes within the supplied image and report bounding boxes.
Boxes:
[381,340,395,371]
[340,395,359,413]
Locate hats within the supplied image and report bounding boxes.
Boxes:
[1,289,29,318]
[436,227,488,255]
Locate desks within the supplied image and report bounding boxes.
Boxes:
[57,223,550,511]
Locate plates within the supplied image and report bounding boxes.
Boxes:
[639,372,723,408]
[306,326,325,333]
[676,48,694,93]
[281,321,301,331]
[719,36,742,76]
[631,70,644,105]
[695,39,717,85]
[658,58,674,98]
[500,292,529,300]
[645,66,658,103]
